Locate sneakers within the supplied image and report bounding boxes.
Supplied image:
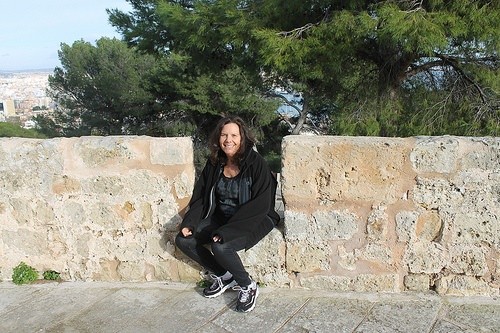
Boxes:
[203,274,238,298]
[235,280,260,313]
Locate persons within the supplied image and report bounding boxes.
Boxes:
[176,116,280,313]
